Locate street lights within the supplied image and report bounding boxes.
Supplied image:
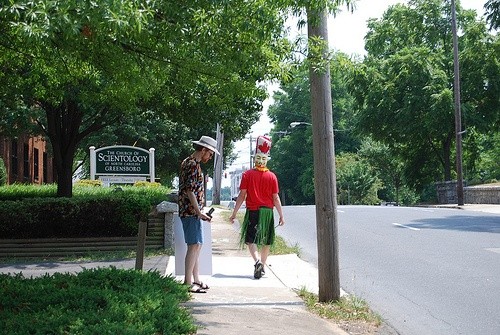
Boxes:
[289,121,312,128]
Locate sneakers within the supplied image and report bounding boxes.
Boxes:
[254,262,265,279]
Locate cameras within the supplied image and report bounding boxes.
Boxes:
[202,207,215,223]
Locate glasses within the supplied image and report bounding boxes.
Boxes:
[207,151,213,157]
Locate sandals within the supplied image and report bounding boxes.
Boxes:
[193,281,210,289]
[187,284,206,293]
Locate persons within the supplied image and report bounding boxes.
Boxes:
[229,136,285,279]
[178,136,221,293]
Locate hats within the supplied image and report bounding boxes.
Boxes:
[191,136,220,155]
